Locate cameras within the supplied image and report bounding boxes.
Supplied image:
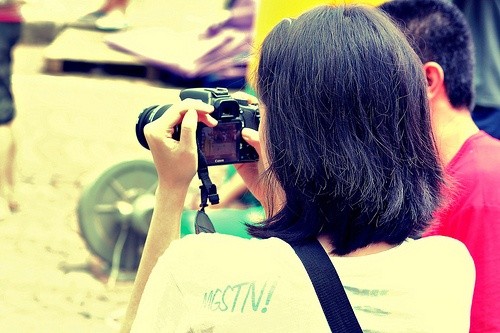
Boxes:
[135,87,261,166]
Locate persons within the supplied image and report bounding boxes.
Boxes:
[378,1,499,333]
[63,0,130,30]
[1,3,24,211]
[122,7,476,333]
[185,166,267,238]
